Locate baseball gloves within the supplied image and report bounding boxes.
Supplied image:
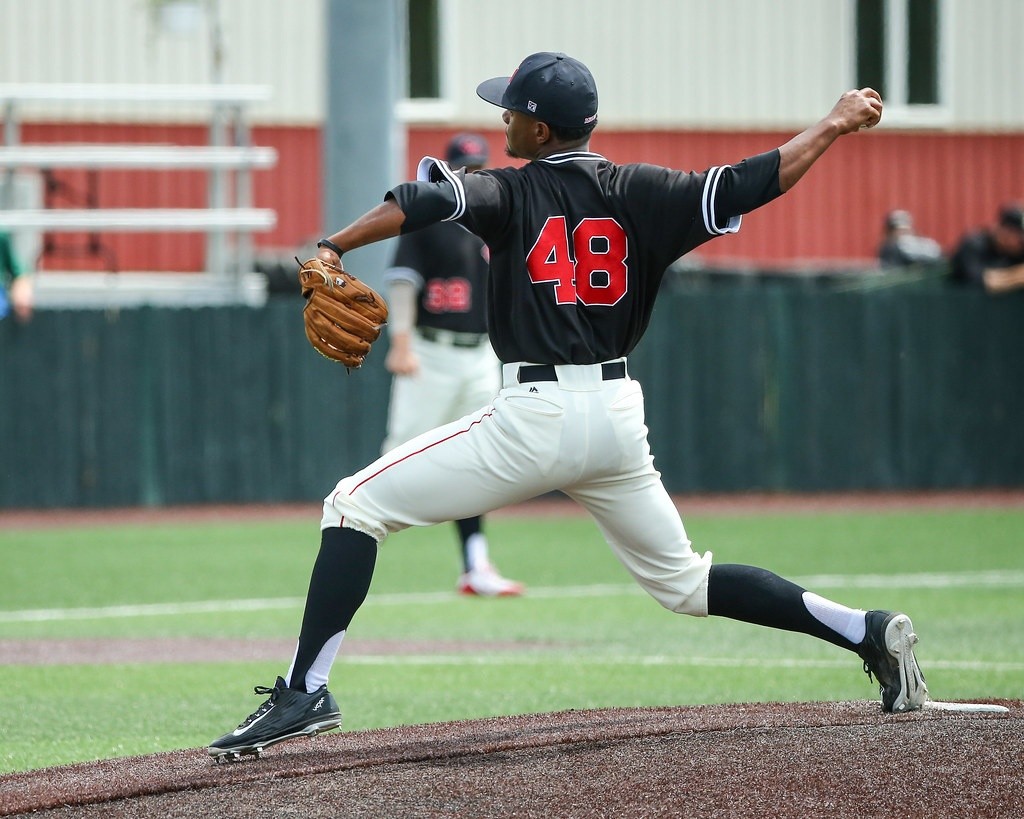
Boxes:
[298,258,390,370]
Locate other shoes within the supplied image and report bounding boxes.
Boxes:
[458,567,523,597]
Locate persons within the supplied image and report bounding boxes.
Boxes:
[207,50,923,762]
[948,202,1024,295]
[879,211,939,269]
[0,230,32,322]
[382,131,522,598]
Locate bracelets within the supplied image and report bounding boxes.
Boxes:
[317,239,343,258]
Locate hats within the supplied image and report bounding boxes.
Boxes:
[885,211,912,230]
[997,205,1024,232]
[476,52,599,128]
[446,134,490,170]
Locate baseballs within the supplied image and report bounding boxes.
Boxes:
[860,97,881,129]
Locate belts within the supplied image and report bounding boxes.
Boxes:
[517,362,626,383]
[420,332,480,347]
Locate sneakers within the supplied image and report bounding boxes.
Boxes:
[207,676,344,766]
[856,609,930,713]
